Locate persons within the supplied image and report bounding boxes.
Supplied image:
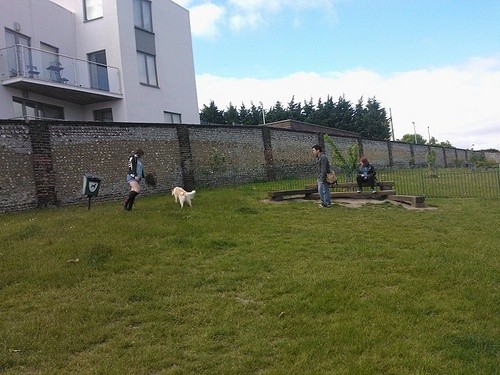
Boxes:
[356,158,378,194]
[311,144,335,208]
[123,149,144,210]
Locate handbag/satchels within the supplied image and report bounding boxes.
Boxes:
[326,169,338,184]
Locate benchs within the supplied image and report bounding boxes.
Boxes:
[267,190,310,201]
[388,194,425,208]
[304,181,396,190]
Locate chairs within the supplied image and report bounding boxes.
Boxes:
[49,70,69,84]
[26,65,40,79]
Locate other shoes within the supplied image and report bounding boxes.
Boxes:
[357,188,363,192]
[371,187,376,193]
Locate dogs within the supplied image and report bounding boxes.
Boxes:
[171,186,197,209]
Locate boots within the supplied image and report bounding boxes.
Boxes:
[127,192,135,211]
[124,190,139,210]
